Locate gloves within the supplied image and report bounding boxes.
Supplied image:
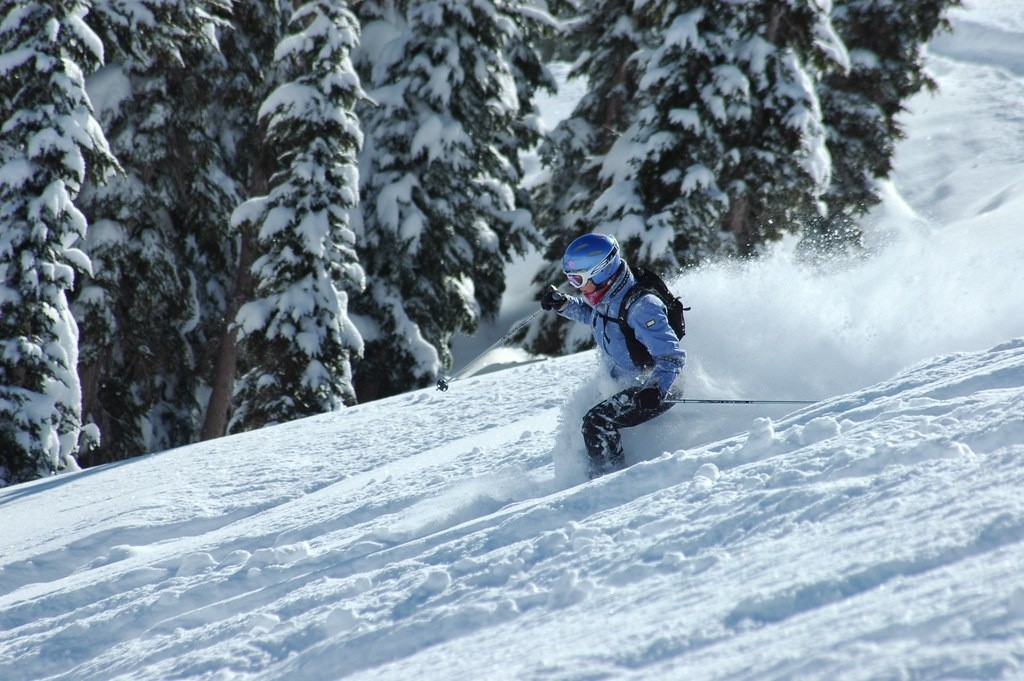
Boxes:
[633,387,661,411]
[541,285,566,312]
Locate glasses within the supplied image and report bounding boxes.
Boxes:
[563,243,620,289]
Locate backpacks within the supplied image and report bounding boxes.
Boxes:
[618,266,691,368]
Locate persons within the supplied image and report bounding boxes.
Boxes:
[541,233,687,479]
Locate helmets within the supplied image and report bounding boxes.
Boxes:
[564,234,620,286]
